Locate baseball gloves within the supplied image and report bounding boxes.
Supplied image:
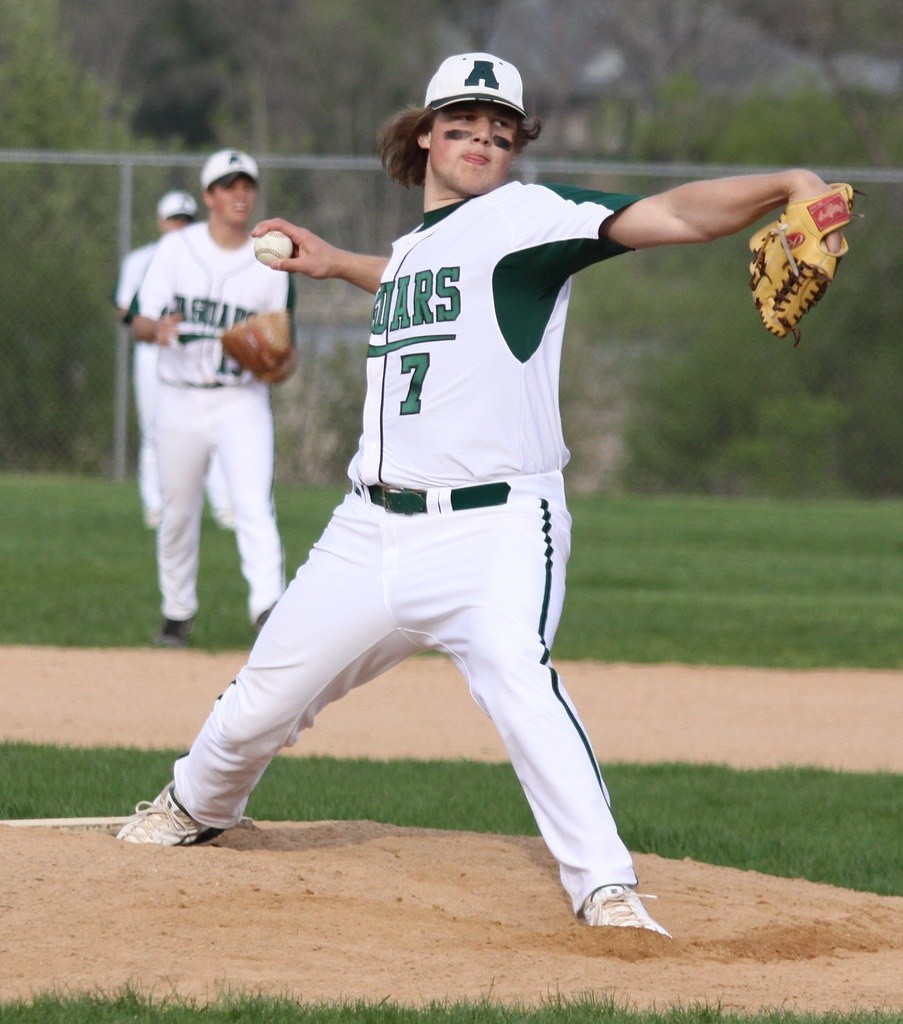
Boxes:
[220,310,295,384]
[746,182,869,350]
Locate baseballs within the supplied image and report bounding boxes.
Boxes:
[254,230,294,267]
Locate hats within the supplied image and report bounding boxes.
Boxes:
[158,191,199,221]
[201,150,259,191]
[423,52,529,119]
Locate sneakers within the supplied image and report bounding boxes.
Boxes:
[580,884,672,936]
[113,778,211,845]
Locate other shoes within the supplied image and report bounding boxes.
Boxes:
[161,617,188,642]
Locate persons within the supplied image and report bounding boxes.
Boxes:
[119,149,296,645]
[116,52,843,940]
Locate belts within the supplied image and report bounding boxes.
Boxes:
[355,481,512,516]
